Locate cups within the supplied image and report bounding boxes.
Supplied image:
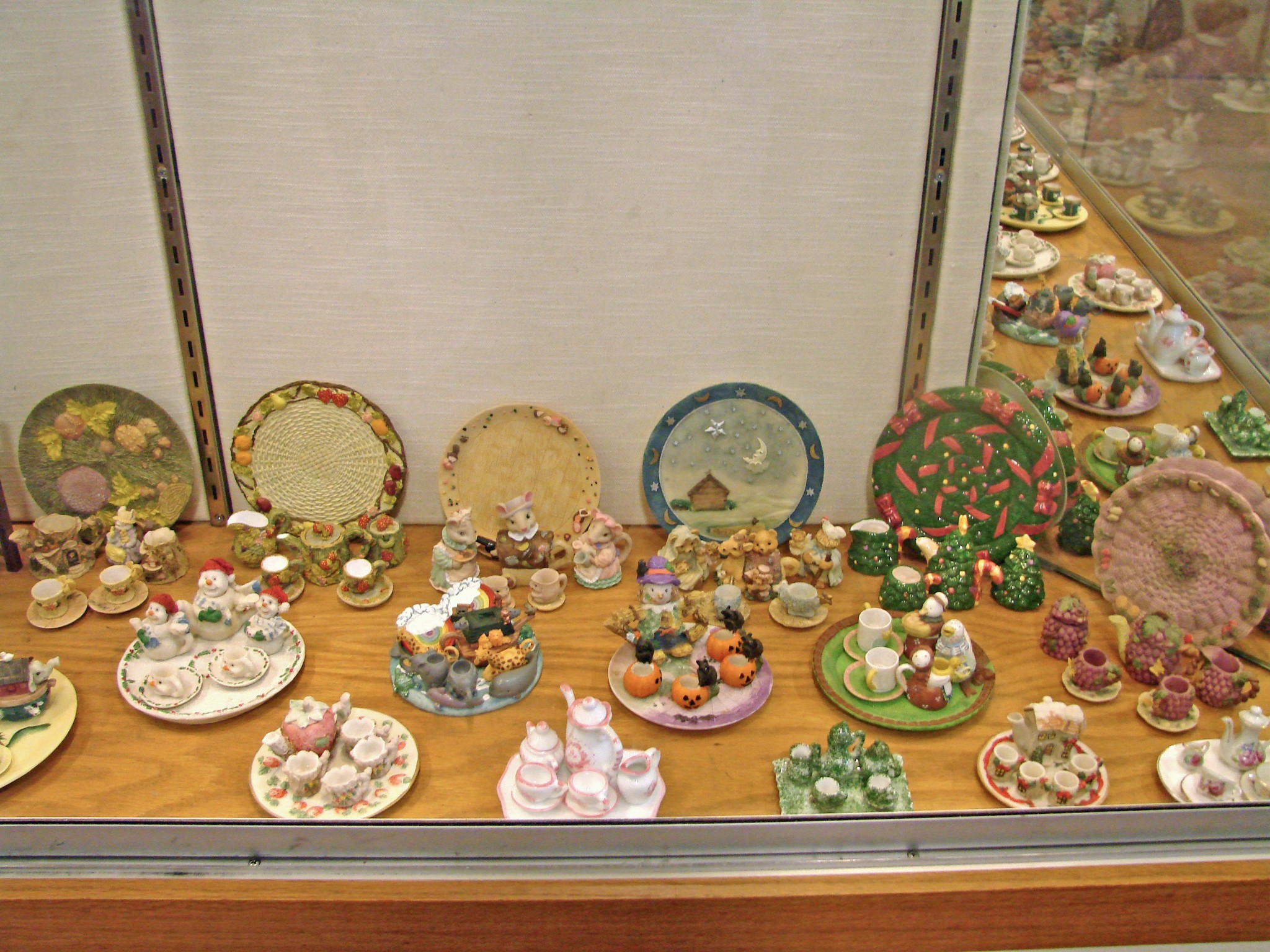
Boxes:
[99,561,143,604]
[141,510,1259,805]
[31,575,79,619]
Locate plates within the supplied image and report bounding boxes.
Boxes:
[772,753,913,815]
[17,383,192,528]
[870,389,1062,564]
[438,402,602,566]
[812,608,995,730]
[0,664,77,789]
[1156,737,1270,803]
[117,618,305,724]
[249,705,421,816]
[1089,468,1270,649]
[497,750,666,821]
[976,730,1107,810]
[644,382,824,548]
[1017,35,1270,316]
[228,382,407,531]
[26,579,149,629]
[606,623,773,730]
[994,118,1270,491]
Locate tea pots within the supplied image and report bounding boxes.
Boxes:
[1110,611,1196,685]
